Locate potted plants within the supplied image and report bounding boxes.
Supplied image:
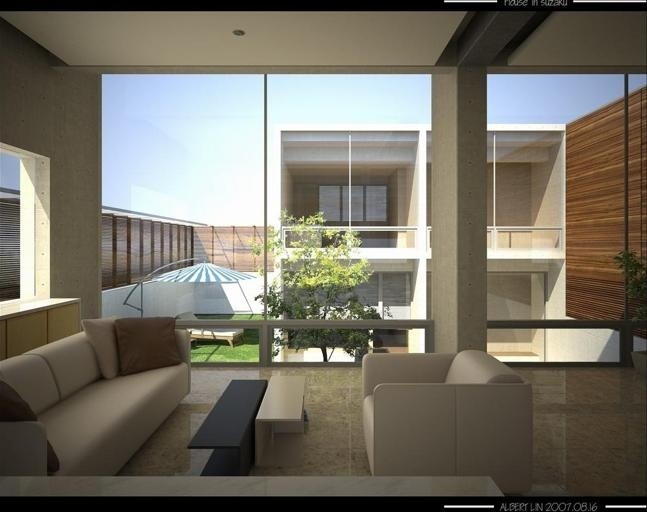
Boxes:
[615,246,646,374]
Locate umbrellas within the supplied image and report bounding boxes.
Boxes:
[120,255,259,317]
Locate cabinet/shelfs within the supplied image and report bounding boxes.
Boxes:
[0,296,81,359]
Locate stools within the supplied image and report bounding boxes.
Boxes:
[189,374,311,477]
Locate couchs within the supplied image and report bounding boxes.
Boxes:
[1,314,194,477]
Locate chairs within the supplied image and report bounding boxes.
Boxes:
[178,310,246,348]
[361,349,534,494]
